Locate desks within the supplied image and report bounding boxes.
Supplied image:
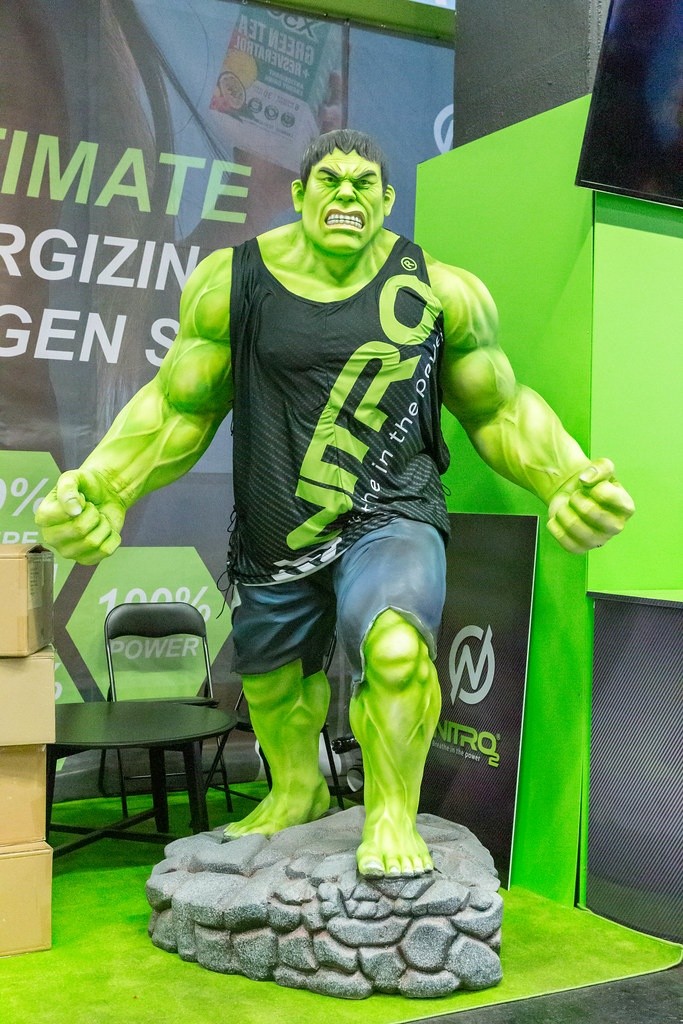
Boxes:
[46,702,238,859]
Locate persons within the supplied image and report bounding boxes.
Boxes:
[31,128,637,878]
[1,2,349,478]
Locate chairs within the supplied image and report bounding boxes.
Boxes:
[189,605,344,827]
[98,602,234,821]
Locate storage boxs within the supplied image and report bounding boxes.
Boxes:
[0,644,56,747]
[0,743,46,848]
[0,544,55,657]
[0,842,52,957]
